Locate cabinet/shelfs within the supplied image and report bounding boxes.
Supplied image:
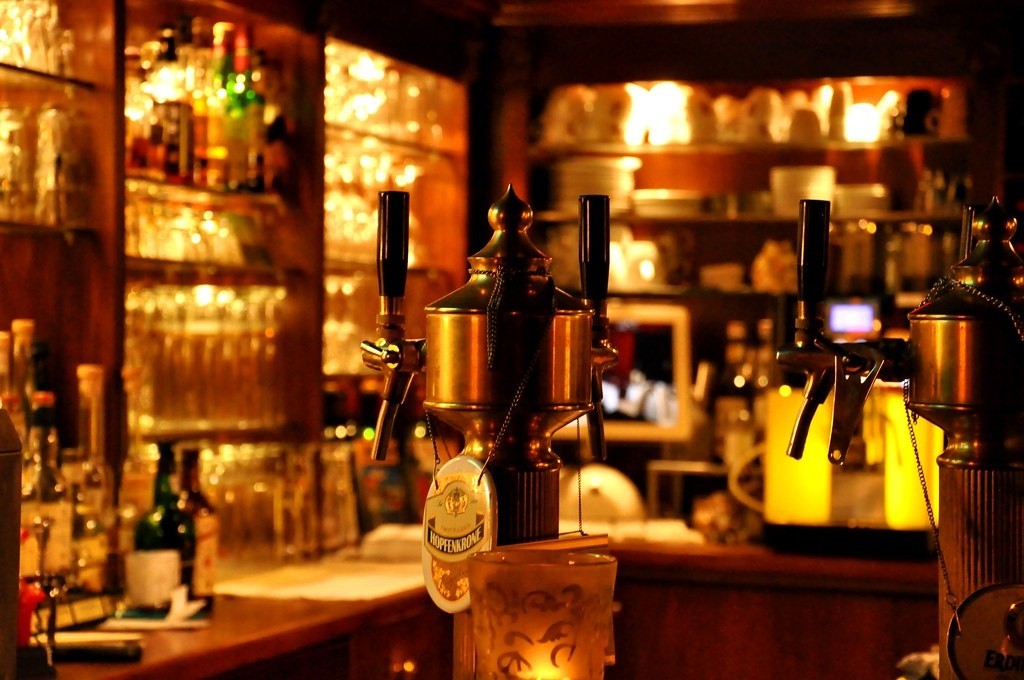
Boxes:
[0,0,471,445]
[541,83,1004,300]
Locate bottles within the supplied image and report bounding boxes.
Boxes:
[124,14,297,194]
[133,438,196,620]
[176,446,219,620]
[0,317,38,500]
[60,364,116,596]
[30,390,74,575]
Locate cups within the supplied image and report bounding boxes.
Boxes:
[882,386,947,531]
[728,386,837,526]
